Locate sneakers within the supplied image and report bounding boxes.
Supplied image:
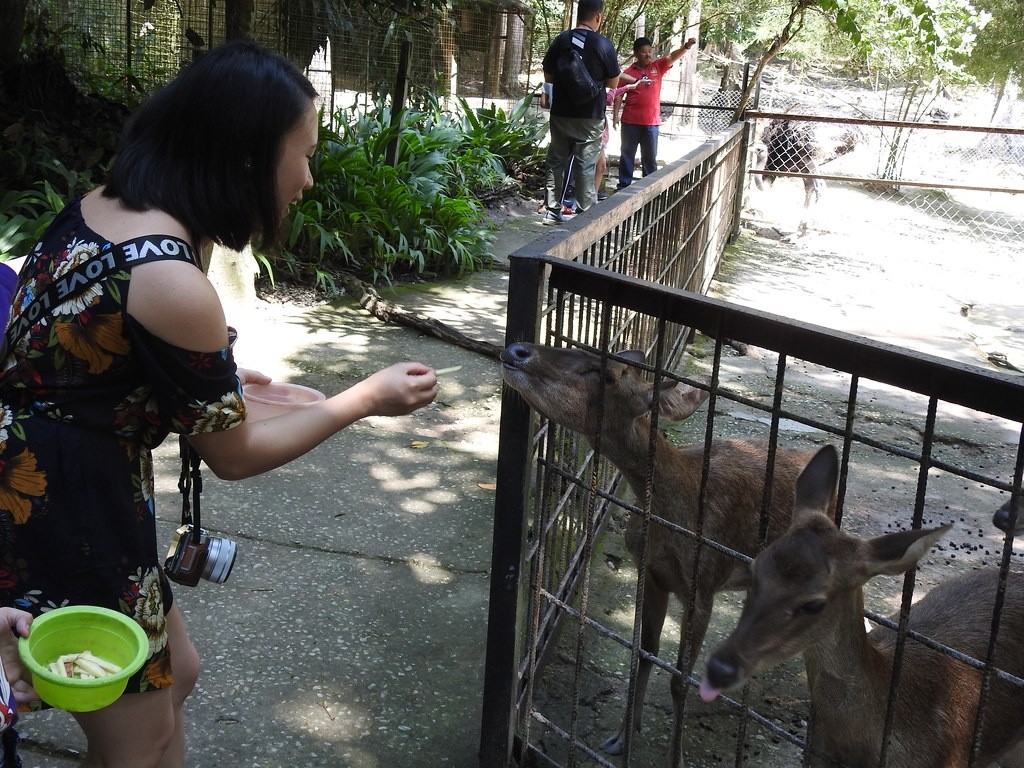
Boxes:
[542,209,569,227]
[537,202,547,214]
[560,204,575,214]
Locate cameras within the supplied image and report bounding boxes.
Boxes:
[164,523,237,587]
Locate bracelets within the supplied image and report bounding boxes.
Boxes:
[685,43,691,50]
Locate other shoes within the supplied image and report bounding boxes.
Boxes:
[597,191,607,201]
[614,185,624,193]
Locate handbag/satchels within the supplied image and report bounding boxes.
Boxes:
[549,29,602,105]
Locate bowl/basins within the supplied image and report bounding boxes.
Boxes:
[17,605,149,713]
[239,383,326,421]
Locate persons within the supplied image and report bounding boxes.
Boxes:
[540,0,696,225]
[0,42,440,768]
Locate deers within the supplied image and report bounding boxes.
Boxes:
[700,443,1024,768]
[497,339,842,768]
